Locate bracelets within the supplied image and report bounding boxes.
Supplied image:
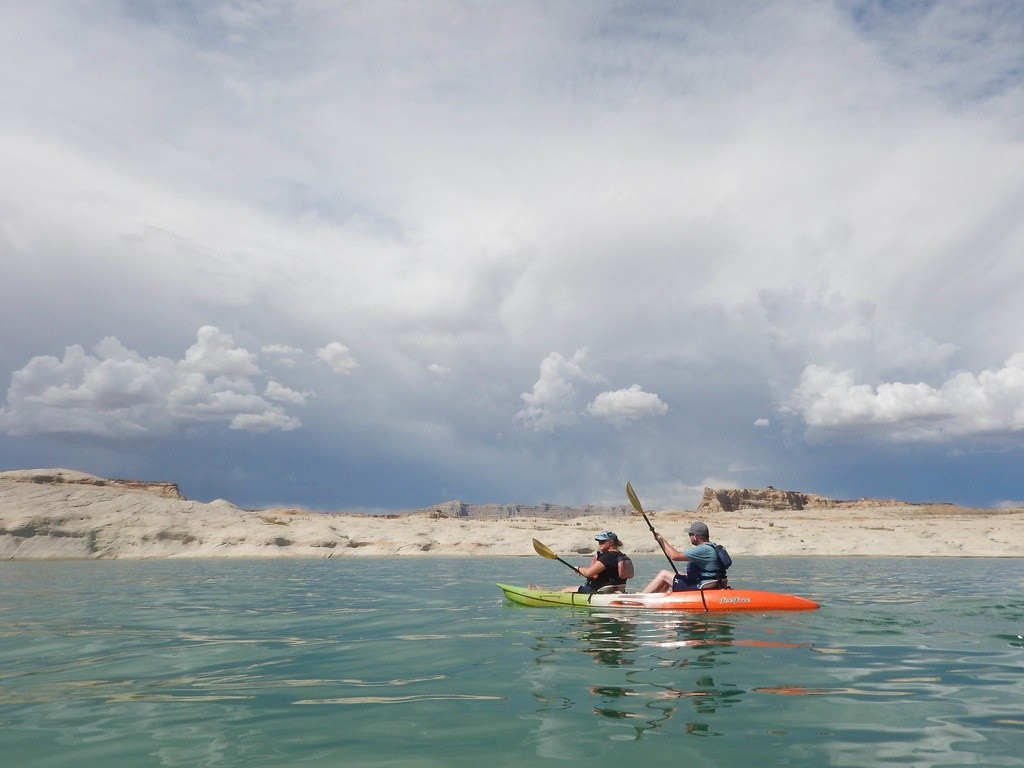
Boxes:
[577,566,584,571]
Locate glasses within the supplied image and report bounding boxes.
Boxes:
[689,533,694,536]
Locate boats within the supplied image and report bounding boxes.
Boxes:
[494,581,823,615]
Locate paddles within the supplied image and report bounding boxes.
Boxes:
[624,480,679,574]
[530,537,587,578]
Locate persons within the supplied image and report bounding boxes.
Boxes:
[643,521,726,594]
[528,531,627,593]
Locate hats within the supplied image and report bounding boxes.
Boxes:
[595,531,618,541]
[684,522,709,536]
